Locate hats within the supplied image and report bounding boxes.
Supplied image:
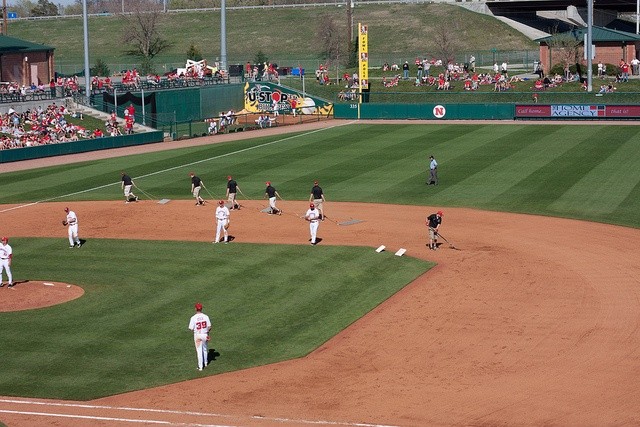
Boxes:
[64,207,68,211]
[219,201,224,203]
[1,237,8,241]
[266,182,271,184]
[190,173,194,175]
[310,204,314,208]
[429,156,433,158]
[195,304,202,310]
[314,181,318,184]
[228,176,232,179]
[437,211,442,216]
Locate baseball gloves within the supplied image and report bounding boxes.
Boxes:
[224,225,229,230]
[62,221,68,226]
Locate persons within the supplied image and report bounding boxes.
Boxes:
[121,170,138,203]
[305,204,320,244]
[204,97,298,135]
[189,171,206,206]
[336,70,370,103]
[425,210,444,251]
[65,207,82,248]
[532,54,640,95]
[213,201,230,244]
[309,179,326,222]
[380,53,527,93]
[226,175,241,211]
[239,58,335,87]
[0,236,14,289]
[0,100,140,151]
[0,70,80,98]
[188,303,212,371]
[91,62,226,93]
[426,155,439,185]
[264,181,283,216]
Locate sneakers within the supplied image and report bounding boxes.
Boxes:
[125,201,129,203]
[204,363,208,366]
[433,244,438,248]
[322,219,324,221]
[197,367,203,371]
[230,208,234,210]
[238,205,240,210]
[310,243,316,246]
[267,211,273,214]
[430,246,434,250]
[212,241,221,243]
[225,241,228,244]
[278,210,282,215]
[195,203,200,205]
[309,239,312,241]
[134,196,138,202]
[0,283,4,287]
[201,201,206,206]
[69,245,74,249]
[7,284,14,288]
[77,244,81,248]
